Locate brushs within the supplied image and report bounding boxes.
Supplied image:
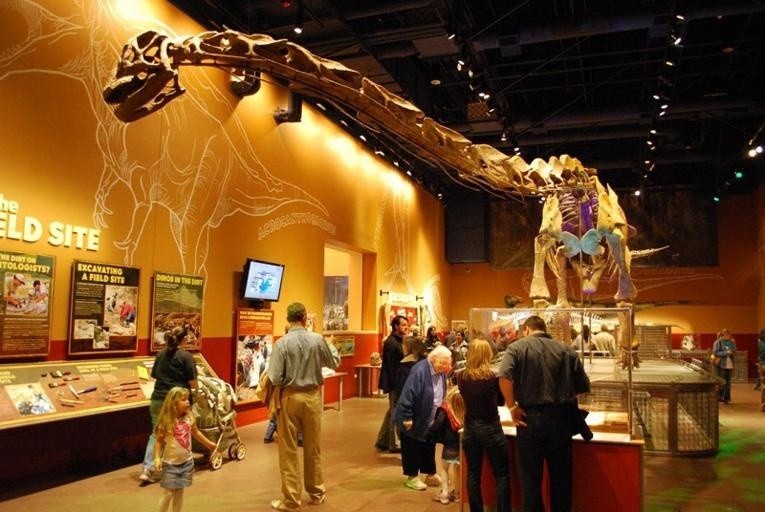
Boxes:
[49,370,80,388]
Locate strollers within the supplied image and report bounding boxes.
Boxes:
[187,373,247,469]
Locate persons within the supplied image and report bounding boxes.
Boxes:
[153,386,216,512]
[264,325,304,447]
[120,301,136,326]
[267,303,341,512]
[391,345,453,489]
[712,326,765,412]
[412,324,527,368]
[139,326,198,483]
[238,336,270,389]
[106,292,118,311]
[570,315,617,358]
[498,315,591,511]
[396,337,428,412]
[431,385,465,504]
[2,273,25,313]
[456,338,512,512]
[376,315,408,452]
[28,279,49,314]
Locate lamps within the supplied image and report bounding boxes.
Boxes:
[441,24,495,112]
[639,1,689,179]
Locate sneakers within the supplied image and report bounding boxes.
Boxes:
[264,438,273,443]
[425,474,443,486]
[406,477,427,490]
[433,488,460,504]
[311,493,325,504]
[375,443,402,453]
[271,500,300,511]
[138,470,156,483]
[297,439,304,446]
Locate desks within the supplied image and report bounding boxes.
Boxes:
[321,371,349,418]
[353,363,386,397]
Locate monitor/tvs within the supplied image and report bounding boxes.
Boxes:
[239,258,285,303]
